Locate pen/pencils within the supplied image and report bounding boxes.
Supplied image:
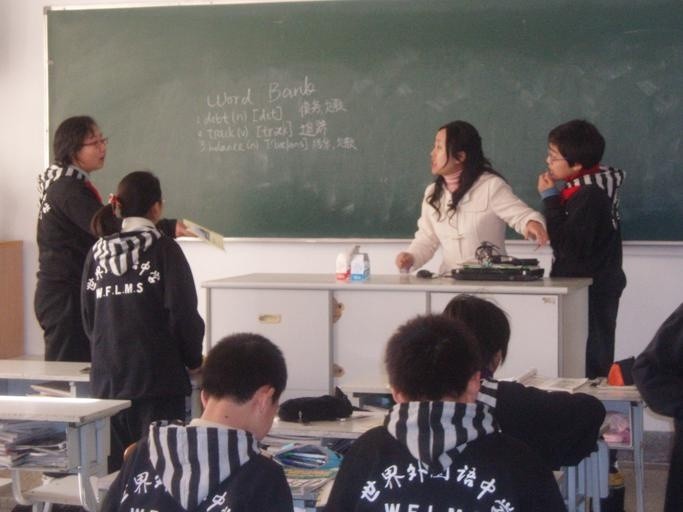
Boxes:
[278,451,327,468]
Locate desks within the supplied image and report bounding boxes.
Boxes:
[0,357,644,512]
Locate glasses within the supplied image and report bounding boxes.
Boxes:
[81,138,106,147]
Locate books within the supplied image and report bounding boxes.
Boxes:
[29,381,70,398]
[0,421,67,468]
[513,367,589,396]
[270,440,344,512]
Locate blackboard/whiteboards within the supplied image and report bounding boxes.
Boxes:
[42,0,683,246]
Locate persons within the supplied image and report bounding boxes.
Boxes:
[632,302,683,511]
[443,291,607,467]
[95,332,295,511]
[78,174,206,472]
[537,118,627,381]
[396,120,550,275]
[34,115,199,361]
[323,311,567,511]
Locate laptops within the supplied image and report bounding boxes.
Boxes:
[452,268,544,281]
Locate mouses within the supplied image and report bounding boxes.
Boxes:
[416,269,434,277]
